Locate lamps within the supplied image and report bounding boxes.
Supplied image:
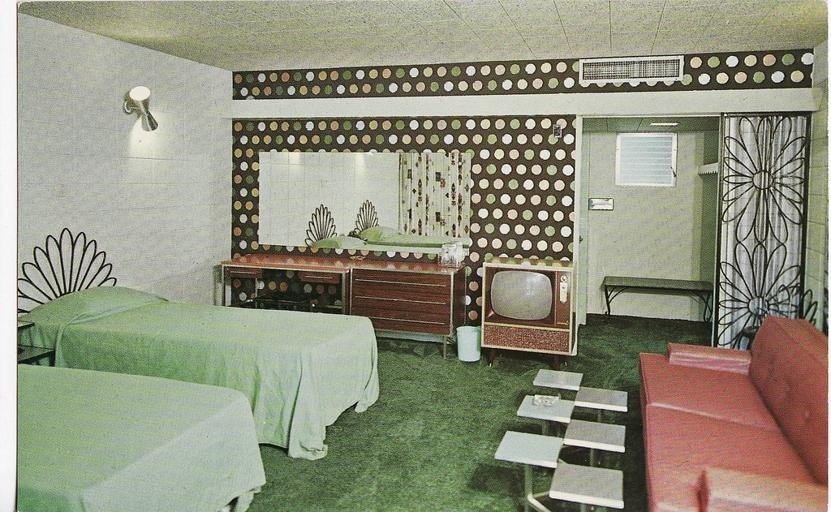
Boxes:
[123,84,159,132]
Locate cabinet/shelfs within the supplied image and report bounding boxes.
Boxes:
[350,258,467,360]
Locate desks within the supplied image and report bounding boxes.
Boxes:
[220,254,359,315]
[601,276,714,326]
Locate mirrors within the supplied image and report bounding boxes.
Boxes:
[258,151,471,256]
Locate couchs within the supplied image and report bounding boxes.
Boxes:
[638,316,829,511]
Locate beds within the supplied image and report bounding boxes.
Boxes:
[18,363,267,510]
[19,284,379,462]
[305,200,470,255]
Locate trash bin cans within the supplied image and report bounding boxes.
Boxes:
[457,325,482,363]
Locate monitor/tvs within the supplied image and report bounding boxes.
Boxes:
[484,257,578,333]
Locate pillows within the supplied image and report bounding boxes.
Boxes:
[21,285,168,325]
